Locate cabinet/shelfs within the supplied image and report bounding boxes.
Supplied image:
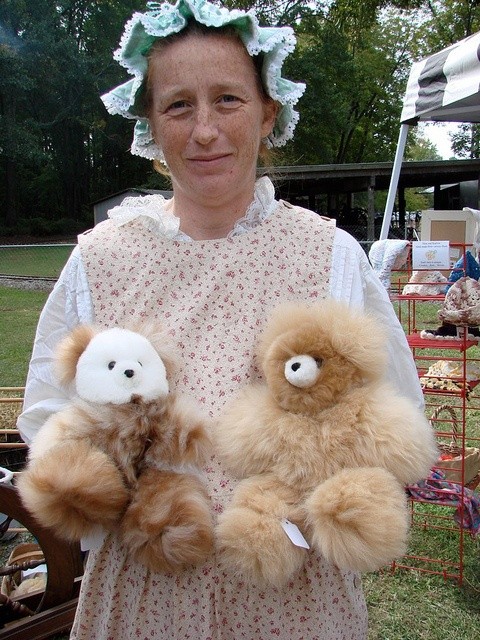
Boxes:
[363,242,480,591]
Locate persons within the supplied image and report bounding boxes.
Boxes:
[16,0,425,640]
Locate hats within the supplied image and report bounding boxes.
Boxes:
[100,0,306,167]
[444,250,480,292]
[439,277,480,325]
[368,238,411,289]
[402,270,448,296]
